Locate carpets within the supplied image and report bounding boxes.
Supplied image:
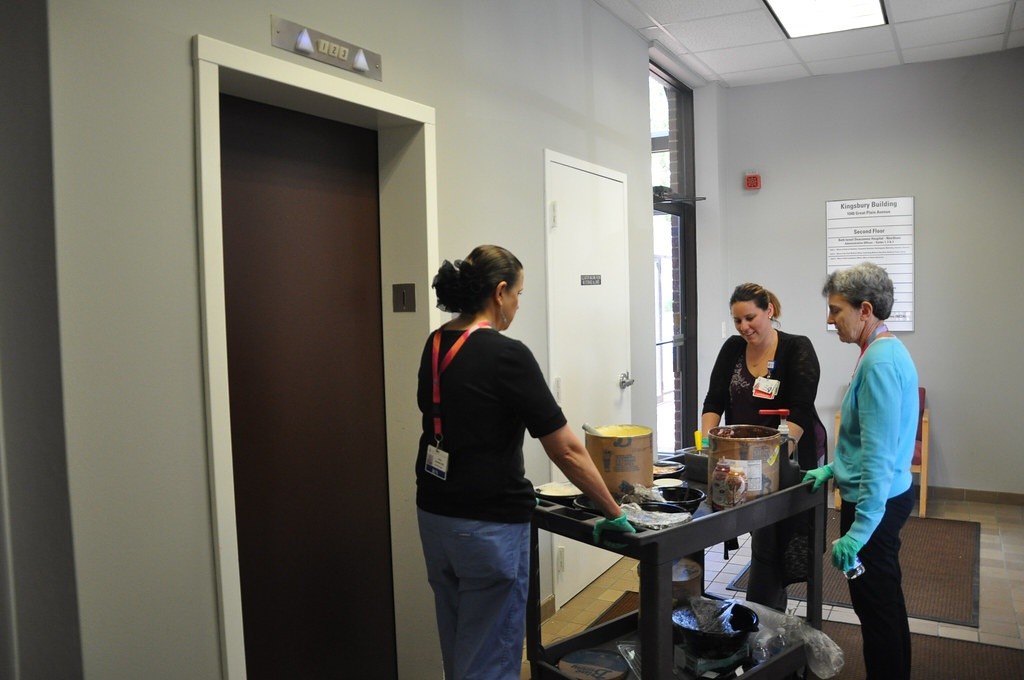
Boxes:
[584,591,1024,680]
[725,505,981,630]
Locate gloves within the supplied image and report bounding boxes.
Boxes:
[801,464,834,496]
[831,535,864,571]
[592,512,635,546]
[701,436,710,447]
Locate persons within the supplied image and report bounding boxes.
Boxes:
[414,244,636,680]
[802,262,920,680]
[702,282,821,612]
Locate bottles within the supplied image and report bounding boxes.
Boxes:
[711,461,732,513]
[766,626,790,658]
[723,466,748,511]
[751,639,767,665]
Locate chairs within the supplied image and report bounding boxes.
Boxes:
[834,386,929,517]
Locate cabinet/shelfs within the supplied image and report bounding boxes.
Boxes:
[525,452,824,680]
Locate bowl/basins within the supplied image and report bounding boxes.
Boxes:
[571,496,623,514]
[534,482,585,507]
[623,485,706,516]
[670,601,760,660]
[653,460,686,481]
